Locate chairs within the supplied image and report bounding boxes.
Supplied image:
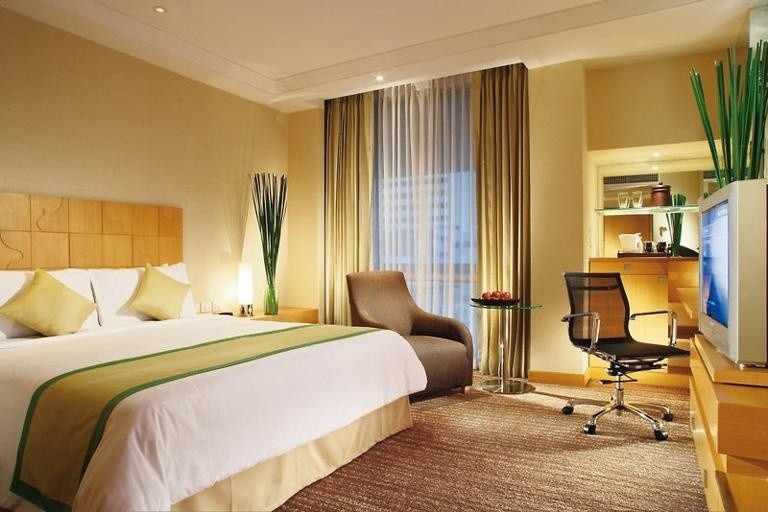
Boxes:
[560,272,690,440]
[346,270,473,400]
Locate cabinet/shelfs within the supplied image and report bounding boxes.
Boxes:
[689,334,768,512]
[588,257,669,374]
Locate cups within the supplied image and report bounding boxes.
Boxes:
[630,190,644,209]
[655,241,666,253]
[199,300,214,315]
[643,240,653,254]
[241,303,254,316]
[617,192,629,208]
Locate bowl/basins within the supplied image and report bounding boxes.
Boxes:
[470,297,521,307]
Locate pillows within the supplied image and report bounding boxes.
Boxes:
[0,262,199,343]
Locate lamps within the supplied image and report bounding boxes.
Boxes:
[238,263,255,318]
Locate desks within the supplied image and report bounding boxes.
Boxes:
[470,303,543,394]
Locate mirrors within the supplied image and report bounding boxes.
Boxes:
[595,154,750,258]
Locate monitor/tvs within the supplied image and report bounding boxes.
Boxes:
[697,178,768,368]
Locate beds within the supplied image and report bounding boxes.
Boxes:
[0,191,427,512]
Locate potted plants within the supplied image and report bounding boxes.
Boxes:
[249,172,288,315]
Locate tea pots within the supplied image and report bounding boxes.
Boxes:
[618,232,642,254]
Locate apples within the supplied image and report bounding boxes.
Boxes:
[483,291,512,300]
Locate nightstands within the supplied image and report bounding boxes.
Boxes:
[233,305,319,324]
